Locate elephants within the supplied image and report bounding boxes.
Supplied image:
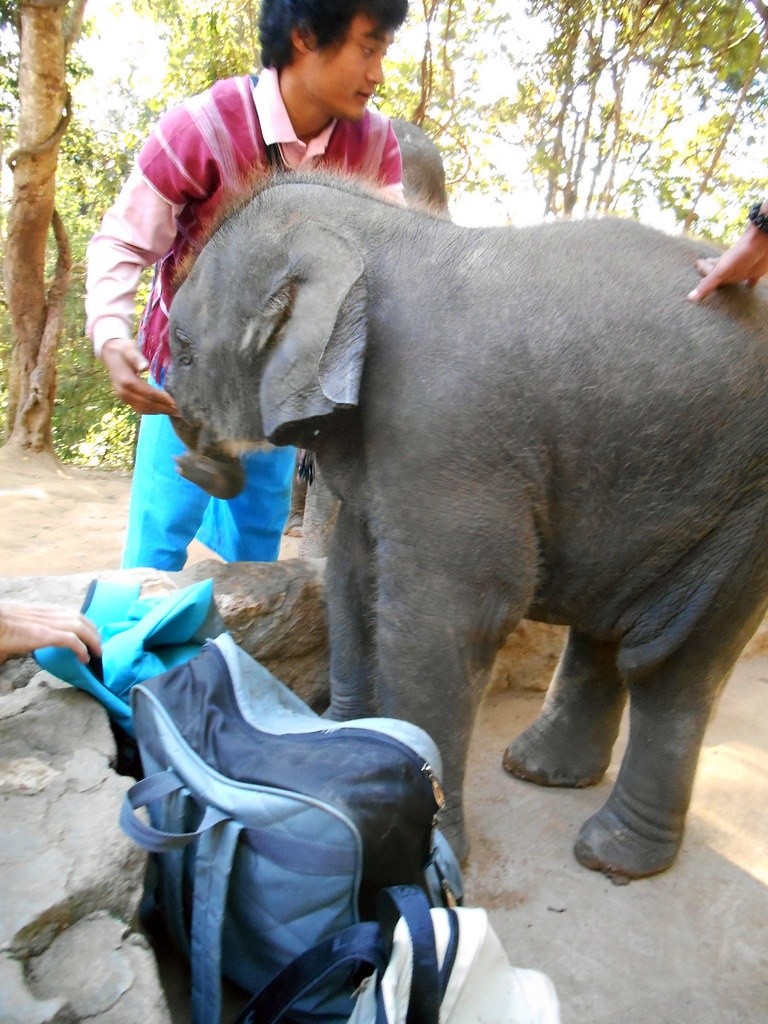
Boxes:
[166,174,767,886]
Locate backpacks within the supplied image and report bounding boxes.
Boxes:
[119,631,463,1024]
[235,883,562,1024]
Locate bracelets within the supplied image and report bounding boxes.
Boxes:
[749,202,768,233]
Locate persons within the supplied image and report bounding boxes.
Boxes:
[85,0,407,572]
[0,600,104,664]
[686,199,768,303]
